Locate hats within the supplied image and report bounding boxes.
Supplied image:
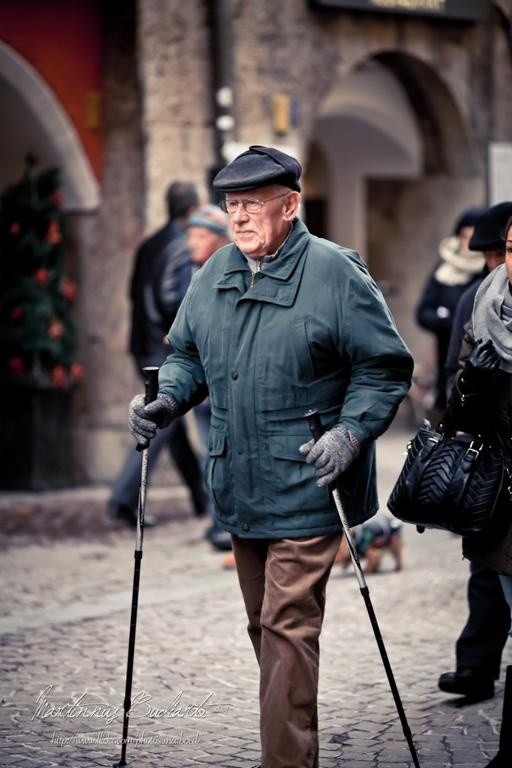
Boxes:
[212,146,302,193]
[469,203,511,254]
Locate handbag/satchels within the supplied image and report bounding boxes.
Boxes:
[386,418,512,539]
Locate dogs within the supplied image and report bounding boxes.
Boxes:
[335,516,404,576]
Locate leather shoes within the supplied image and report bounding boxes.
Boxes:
[438,668,495,699]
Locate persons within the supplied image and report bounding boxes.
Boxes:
[445,212,512,768]
[105,180,212,529]
[168,204,234,549]
[416,206,488,413]
[128,147,415,768]
[438,201,511,698]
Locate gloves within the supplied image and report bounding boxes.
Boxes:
[299,424,362,487]
[129,392,178,444]
[458,338,501,393]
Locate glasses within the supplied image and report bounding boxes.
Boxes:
[218,191,290,213]
